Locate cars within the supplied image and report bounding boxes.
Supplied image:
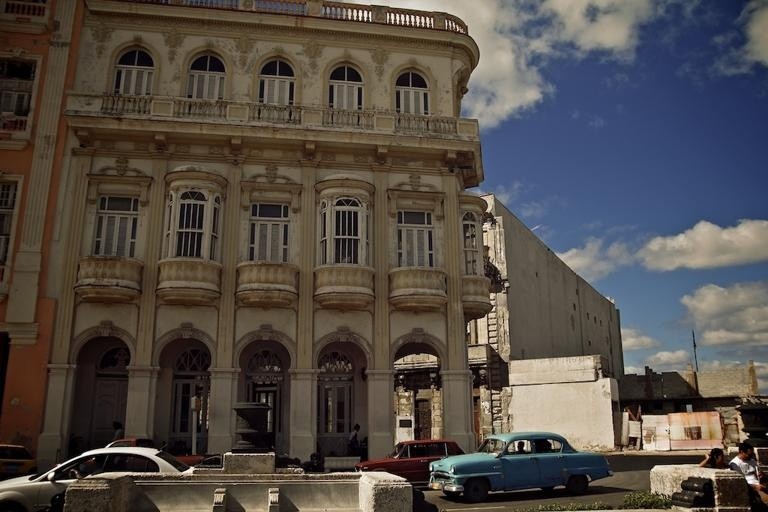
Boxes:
[428,432,610,500]
[0,445,37,479]
[355,439,466,482]
[1,447,194,511]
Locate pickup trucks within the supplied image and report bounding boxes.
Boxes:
[86,439,203,469]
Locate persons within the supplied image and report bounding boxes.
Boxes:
[513,441,525,453]
[344,423,361,456]
[110,419,125,441]
[730,441,768,512]
[69,467,84,480]
[699,448,734,470]
